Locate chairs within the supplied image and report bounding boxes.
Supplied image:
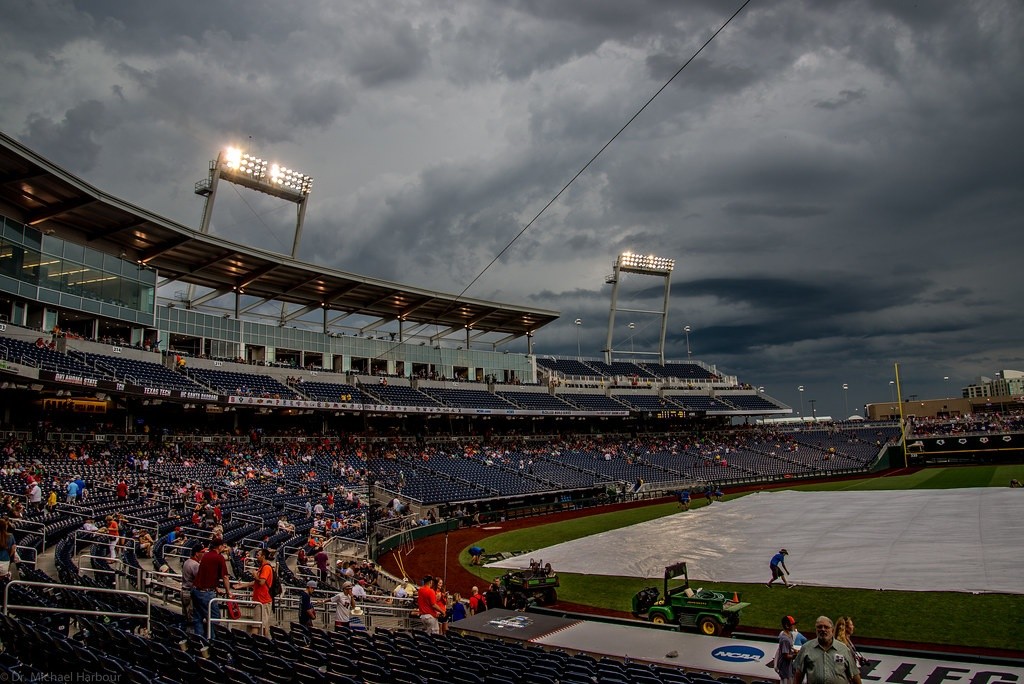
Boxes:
[0,333,1024,684]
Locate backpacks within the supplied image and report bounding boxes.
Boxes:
[261,563,282,597]
[474,596,486,614]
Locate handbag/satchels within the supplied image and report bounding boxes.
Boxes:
[110,537,116,541]
[388,498,394,508]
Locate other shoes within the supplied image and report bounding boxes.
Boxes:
[765,585,772,588]
[786,584,793,589]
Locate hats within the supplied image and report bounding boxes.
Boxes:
[175,527,183,531]
[349,489,352,492]
[31,482,37,485]
[342,581,353,590]
[307,581,317,587]
[69,475,80,481]
[210,538,224,546]
[336,560,367,564]
[781,549,788,554]
[351,607,363,615]
[782,616,798,626]
[472,586,478,591]
[120,479,123,482]
[359,580,366,584]
[403,578,409,581]
[424,574,434,582]
[191,543,208,553]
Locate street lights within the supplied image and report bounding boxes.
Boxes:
[842,384,849,418]
[994,372,1004,416]
[758,386,765,425]
[683,326,691,361]
[574,318,582,357]
[944,376,953,417]
[889,380,897,419]
[798,385,804,423]
[628,323,635,359]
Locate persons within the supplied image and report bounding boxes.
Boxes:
[765,549,794,589]
[0,325,1024,684]
[468,547,485,567]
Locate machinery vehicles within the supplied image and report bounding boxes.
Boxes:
[500,559,560,606]
[631,562,752,636]
